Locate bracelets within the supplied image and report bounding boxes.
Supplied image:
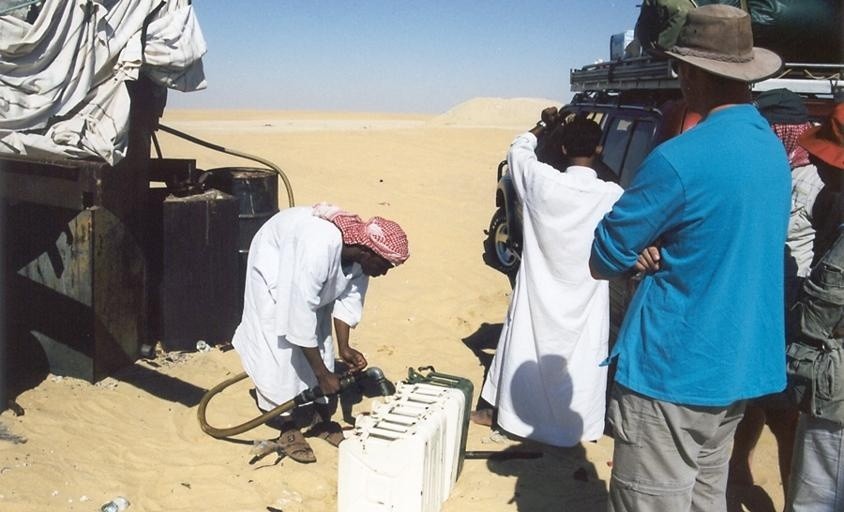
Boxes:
[537,120,546,128]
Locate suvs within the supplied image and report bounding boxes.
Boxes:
[483,2,842,375]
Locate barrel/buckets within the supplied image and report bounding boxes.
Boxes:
[336,366,474,512]
[202,166,281,325]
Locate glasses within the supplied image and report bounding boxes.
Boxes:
[671,60,685,73]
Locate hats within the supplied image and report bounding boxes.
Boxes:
[797,103,844,170]
[664,5,782,81]
[756,88,808,124]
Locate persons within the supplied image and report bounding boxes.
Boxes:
[230,201,410,463]
[586,4,788,510]
[466,106,625,446]
[783,101,844,510]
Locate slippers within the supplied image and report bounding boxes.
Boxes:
[275,429,315,462]
[308,420,345,446]
